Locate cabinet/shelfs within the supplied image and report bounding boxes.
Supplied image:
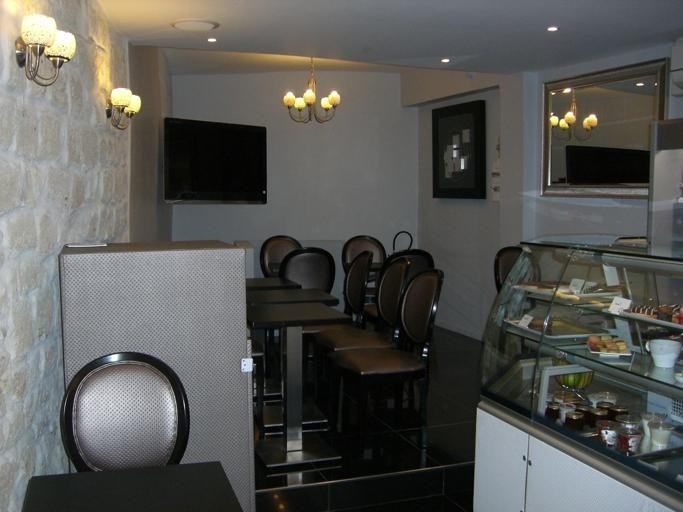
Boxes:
[469,237,683,512]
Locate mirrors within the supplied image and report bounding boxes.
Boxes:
[541,56,670,197]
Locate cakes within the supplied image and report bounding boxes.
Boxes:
[632,304,683,325]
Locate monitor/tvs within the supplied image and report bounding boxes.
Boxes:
[161,117,268,204]
[567,147,648,184]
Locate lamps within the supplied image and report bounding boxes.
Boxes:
[281,56,341,125]
[11,12,78,90]
[549,86,599,143]
[104,86,143,132]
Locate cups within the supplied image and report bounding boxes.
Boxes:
[648,339,681,368]
[640,410,673,445]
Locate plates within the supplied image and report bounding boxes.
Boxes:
[585,344,631,360]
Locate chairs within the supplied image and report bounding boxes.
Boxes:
[493,244,542,356]
[56,349,192,471]
[245,235,444,469]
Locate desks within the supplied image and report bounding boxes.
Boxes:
[20,458,243,511]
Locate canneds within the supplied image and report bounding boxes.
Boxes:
[545,402,628,430]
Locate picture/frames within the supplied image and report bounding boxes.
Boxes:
[431,99,487,201]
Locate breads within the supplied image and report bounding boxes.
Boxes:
[587,335,627,353]
